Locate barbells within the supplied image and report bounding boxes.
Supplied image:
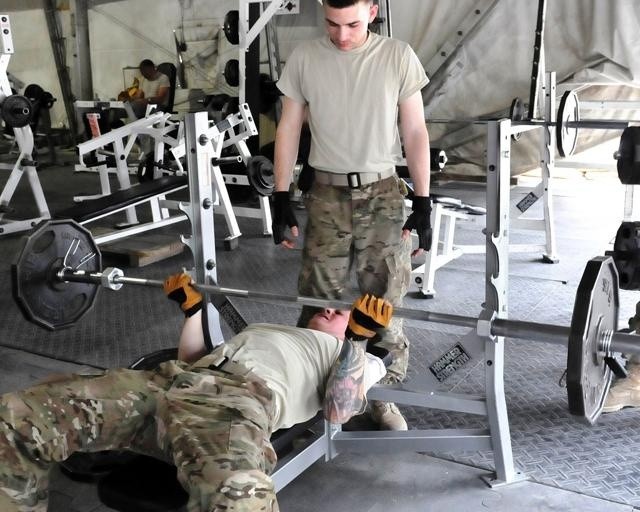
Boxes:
[425,86,631,159]
[11,217,640,427]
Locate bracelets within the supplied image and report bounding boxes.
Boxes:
[148,97,153,104]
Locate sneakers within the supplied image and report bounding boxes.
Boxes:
[602,365,640,413]
[369,401,408,431]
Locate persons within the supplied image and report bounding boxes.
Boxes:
[130,59,170,118]
[268,0,432,431]
[0,272,393,512]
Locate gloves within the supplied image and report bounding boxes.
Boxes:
[191,355,266,391]
[345,293,393,341]
[164,274,203,318]
[314,167,395,188]
